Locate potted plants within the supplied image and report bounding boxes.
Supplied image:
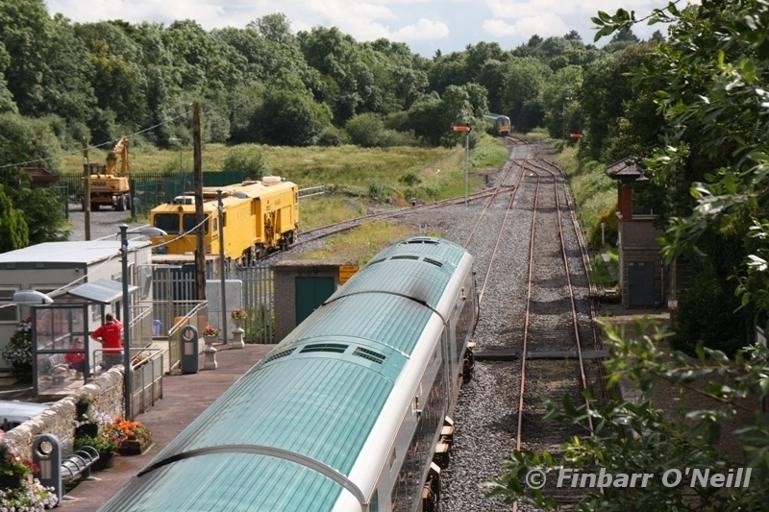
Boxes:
[73,393,117,468]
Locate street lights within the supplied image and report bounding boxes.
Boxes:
[0,291,53,314]
[118,223,168,420]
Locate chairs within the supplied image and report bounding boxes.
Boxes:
[48,352,71,385]
[60,440,100,500]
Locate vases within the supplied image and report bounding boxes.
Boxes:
[203,335,219,369]
[121,431,152,454]
[232,320,244,348]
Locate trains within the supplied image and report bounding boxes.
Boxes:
[150,175,298,276]
[93,236,478,510]
[483,113,510,137]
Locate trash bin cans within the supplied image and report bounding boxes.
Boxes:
[32,433,62,505]
[179,325,199,374]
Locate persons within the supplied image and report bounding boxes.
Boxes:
[66,338,92,377]
[91,314,124,368]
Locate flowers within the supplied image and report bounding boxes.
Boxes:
[112,416,145,437]
[203,325,218,336]
[231,308,247,319]
[0,429,59,512]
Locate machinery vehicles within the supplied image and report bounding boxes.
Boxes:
[77,136,133,211]
